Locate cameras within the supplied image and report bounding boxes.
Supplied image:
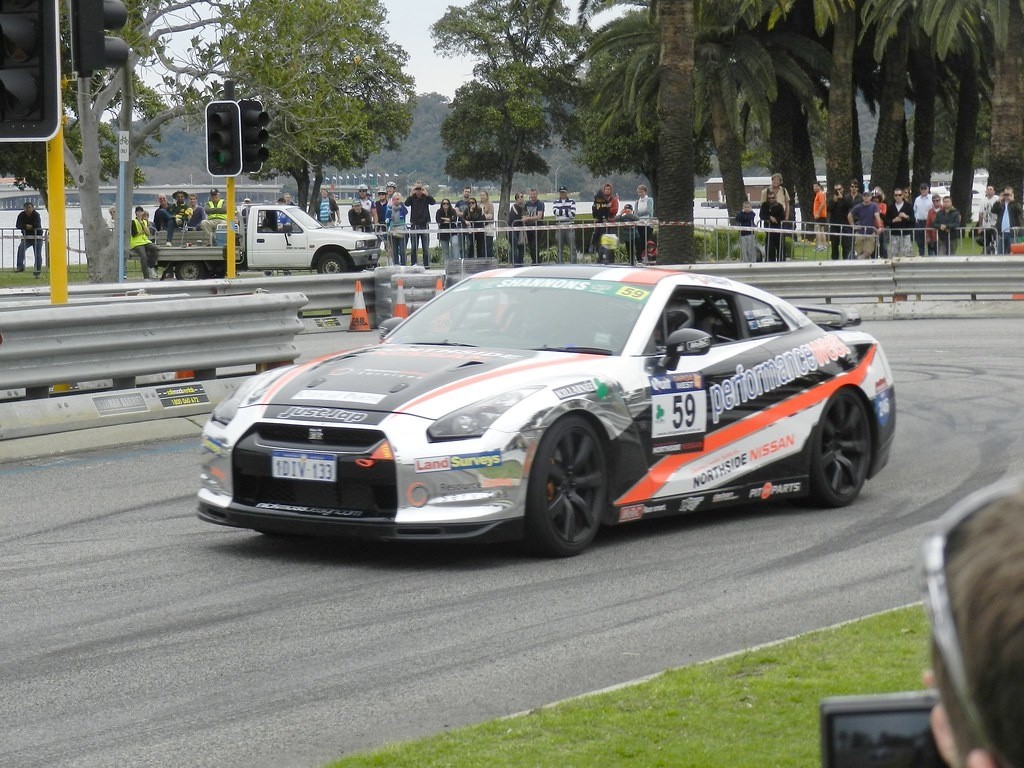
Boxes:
[415,187,422,190]
[1003,194,1009,196]
[623,210,629,213]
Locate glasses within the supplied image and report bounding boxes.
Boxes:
[914,477,1019,745]
[517,196,523,198]
[442,203,449,205]
[768,183,942,206]
[468,202,475,204]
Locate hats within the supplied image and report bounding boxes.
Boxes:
[172,190,189,199]
[243,198,251,202]
[920,183,927,188]
[863,190,871,196]
[595,190,604,199]
[135,207,143,211]
[210,188,220,194]
[559,186,568,193]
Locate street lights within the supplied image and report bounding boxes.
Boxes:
[399,170,417,197]
[550,164,562,193]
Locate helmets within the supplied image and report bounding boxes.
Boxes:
[377,188,387,194]
[366,189,372,196]
[658,300,697,330]
[352,199,361,205]
[356,184,368,190]
[385,182,396,190]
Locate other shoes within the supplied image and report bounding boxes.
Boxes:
[148,268,157,278]
[15,269,23,273]
[33,274,40,278]
[814,246,826,251]
[166,241,172,247]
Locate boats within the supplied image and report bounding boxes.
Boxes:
[930,185,986,212]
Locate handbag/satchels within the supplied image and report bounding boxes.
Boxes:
[518,231,529,246]
[391,226,405,239]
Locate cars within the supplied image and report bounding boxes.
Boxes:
[193,263,898,561]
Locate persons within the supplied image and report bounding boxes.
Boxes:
[14,202,43,279]
[614,204,640,266]
[153,188,227,247]
[654,297,693,346]
[735,201,755,263]
[506,188,545,267]
[919,482,1024,768]
[348,180,408,269]
[827,179,961,260]
[759,173,790,262]
[813,182,827,252]
[129,206,159,282]
[633,184,653,261]
[552,187,577,264]
[991,186,1023,255]
[242,198,251,224]
[436,187,495,262]
[317,188,341,226]
[977,185,1000,255]
[404,181,435,269]
[593,184,618,264]
[107,206,127,279]
[277,193,295,224]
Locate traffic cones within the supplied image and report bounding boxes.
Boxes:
[346,279,372,332]
[431,279,451,332]
[496,291,509,326]
[394,279,408,319]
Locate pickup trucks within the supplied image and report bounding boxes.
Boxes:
[156,204,379,274]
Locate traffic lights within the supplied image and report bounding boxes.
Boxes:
[205,101,242,177]
[70,0,128,78]
[237,99,270,173]
[0,0,61,142]
